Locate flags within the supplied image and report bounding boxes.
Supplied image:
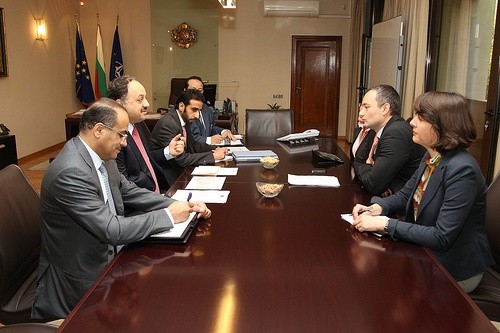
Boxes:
[95,24,108,100]
[73,17,95,107]
[108,22,125,89]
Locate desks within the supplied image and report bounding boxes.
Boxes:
[54,139,500,333]
[65,112,233,140]
[0,135,18,171]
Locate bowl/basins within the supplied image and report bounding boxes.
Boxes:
[255,182,284,198]
[260,160,280,169]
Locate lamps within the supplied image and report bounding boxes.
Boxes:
[35,18,48,40]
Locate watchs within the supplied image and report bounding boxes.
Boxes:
[384,219,389,235]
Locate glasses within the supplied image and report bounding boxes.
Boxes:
[95,123,128,141]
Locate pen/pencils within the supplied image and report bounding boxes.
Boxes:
[187,192,192,202]
[211,150,216,152]
[217,133,225,140]
[349,210,365,217]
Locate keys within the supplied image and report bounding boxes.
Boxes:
[483,112,492,132]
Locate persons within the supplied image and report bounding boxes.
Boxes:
[348,84,424,197]
[176,75,237,148]
[30,97,211,319]
[353,91,496,293]
[144,87,229,192]
[105,74,187,223]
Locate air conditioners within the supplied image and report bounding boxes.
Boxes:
[264,0,319,18]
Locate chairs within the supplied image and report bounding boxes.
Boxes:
[0,164,60,333]
[157,78,186,113]
[245,109,295,144]
[467,170,500,322]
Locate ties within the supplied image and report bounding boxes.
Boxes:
[369,136,379,166]
[132,127,160,194]
[182,127,187,146]
[98,163,117,216]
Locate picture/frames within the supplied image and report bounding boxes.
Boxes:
[0,7,9,78]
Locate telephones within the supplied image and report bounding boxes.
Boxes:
[311,149,345,167]
